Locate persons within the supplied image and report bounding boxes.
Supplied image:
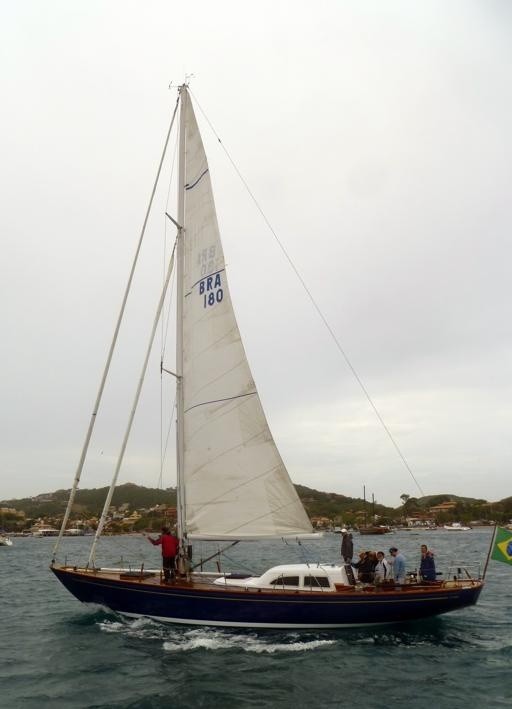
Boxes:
[339,528,357,585]
[418,543,436,582]
[389,547,407,591]
[146,526,181,584]
[350,550,391,592]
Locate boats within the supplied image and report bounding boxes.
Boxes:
[0,525,87,548]
[322,521,477,534]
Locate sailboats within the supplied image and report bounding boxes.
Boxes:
[356,484,392,535]
[40,77,488,631]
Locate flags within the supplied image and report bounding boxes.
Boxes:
[491,525,512,567]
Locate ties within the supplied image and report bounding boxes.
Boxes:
[383,562,386,579]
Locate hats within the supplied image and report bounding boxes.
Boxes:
[340,527,348,533]
